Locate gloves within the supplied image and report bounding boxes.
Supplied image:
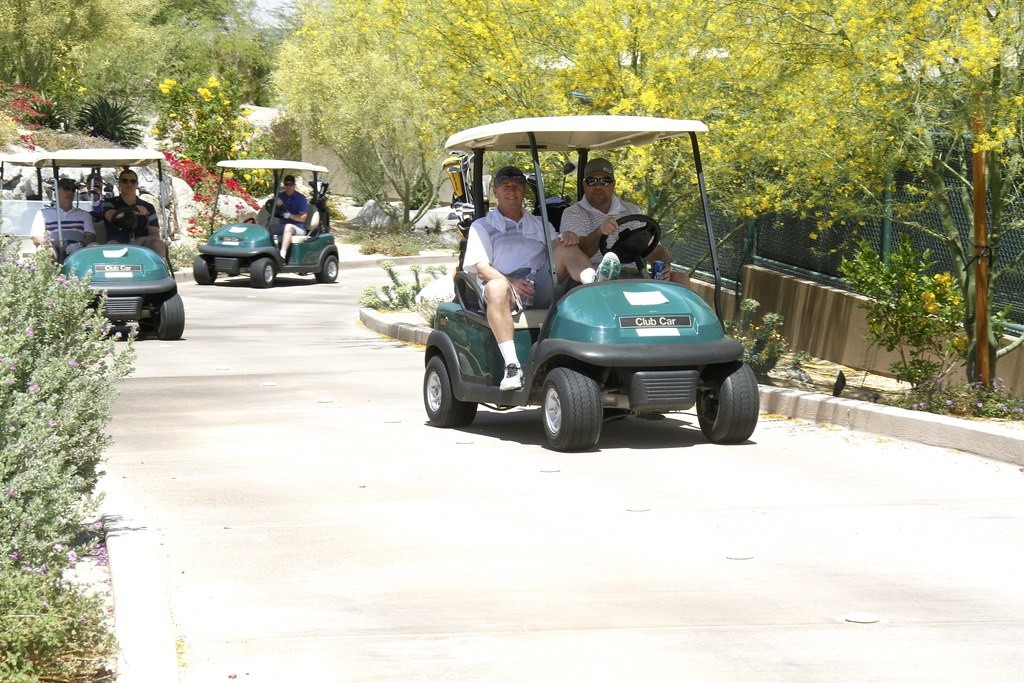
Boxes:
[282,211,291,218]
[66,243,83,254]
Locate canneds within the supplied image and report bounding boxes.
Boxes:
[653,260,665,279]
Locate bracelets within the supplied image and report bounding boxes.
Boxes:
[78,241,85,247]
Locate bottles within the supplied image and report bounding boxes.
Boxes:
[522,268,537,306]
[273,235,278,245]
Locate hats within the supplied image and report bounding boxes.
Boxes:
[58,179,79,190]
[285,176,294,181]
[584,158,613,181]
[494,166,526,198]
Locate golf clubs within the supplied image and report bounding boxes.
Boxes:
[523,172,544,204]
[75,182,86,208]
[308,179,329,211]
[561,161,577,198]
[441,150,493,229]
[43,177,61,209]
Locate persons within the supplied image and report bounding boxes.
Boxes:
[560,158,690,292]
[30,170,167,266]
[265,175,308,266]
[463,165,621,391]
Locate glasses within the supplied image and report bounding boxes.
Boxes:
[496,174,522,180]
[285,184,293,186]
[119,177,137,183]
[585,177,612,186]
[64,186,75,193]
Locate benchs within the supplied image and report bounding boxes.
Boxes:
[257,204,320,244]
[88,211,108,245]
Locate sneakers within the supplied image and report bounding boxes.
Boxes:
[500,363,523,391]
[592,252,621,282]
